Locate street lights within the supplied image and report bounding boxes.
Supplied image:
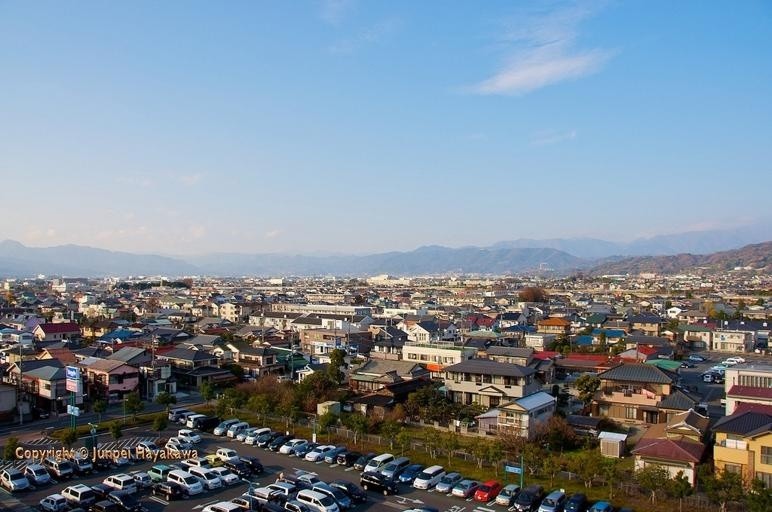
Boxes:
[437,328,448,341]
[261,308,266,343]
[290,347,297,379]
[152,335,161,369]
[343,318,351,355]
[9,343,32,425]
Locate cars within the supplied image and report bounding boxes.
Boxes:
[702,355,747,382]
[688,355,704,362]
[555,355,563,359]
[681,361,694,368]
[291,345,301,350]
[245,375,257,383]
[357,353,366,358]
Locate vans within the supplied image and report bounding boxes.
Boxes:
[277,376,293,383]
